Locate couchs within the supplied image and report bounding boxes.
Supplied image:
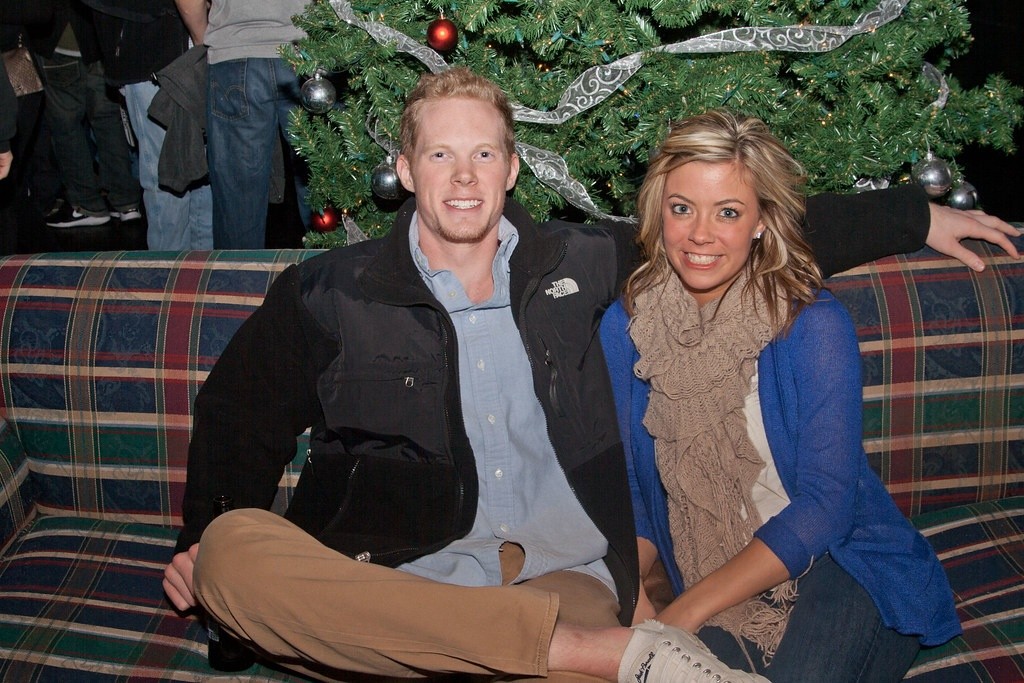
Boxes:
[1,221,1024,683]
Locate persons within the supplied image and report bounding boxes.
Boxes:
[600,108,962,683]
[0,0,319,254]
[163,70,1024,683]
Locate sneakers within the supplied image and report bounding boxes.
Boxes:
[109,206,141,222]
[625,618,771,683]
[45,204,110,227]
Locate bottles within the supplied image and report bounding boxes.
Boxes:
[207,495,255,672]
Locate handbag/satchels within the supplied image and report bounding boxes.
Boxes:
[2,47,43,96]
